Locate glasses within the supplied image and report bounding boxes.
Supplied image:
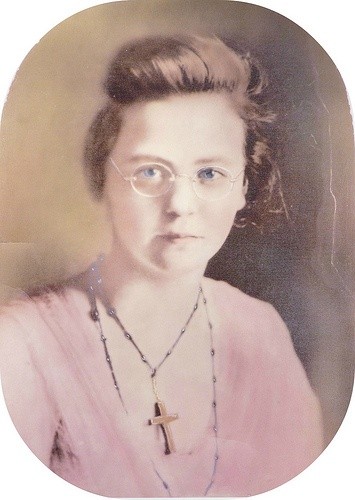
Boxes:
[108,154,246,203]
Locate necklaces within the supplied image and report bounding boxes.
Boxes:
[90,251,218,499]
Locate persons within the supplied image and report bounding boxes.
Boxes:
[1,34,324,500]
